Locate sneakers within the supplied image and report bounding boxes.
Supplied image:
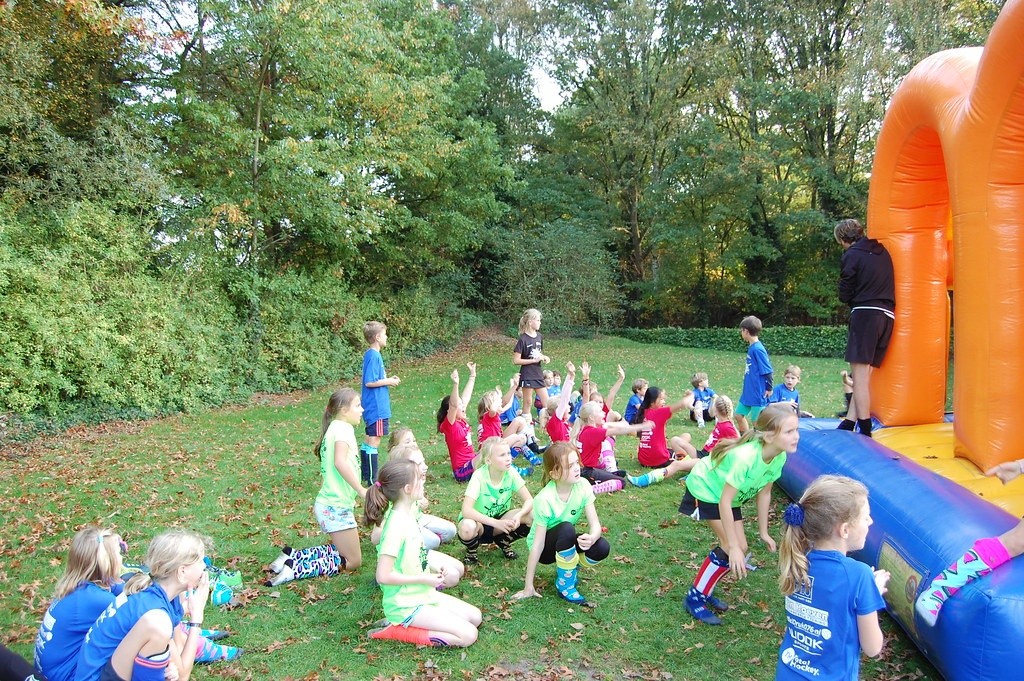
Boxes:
[204,566,219,580]
[208,570,243,590]
[211,580,233,607]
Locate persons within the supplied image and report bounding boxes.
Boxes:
[735,315,815,439]
[834,219,895,438]
[457,436,536,561]
[514,308,552,437]
[363,427,482,647]
[775,475,891,681]
[361,322,401,486]
[436,360,741,494]
[679,402,800,623]
[33,526,245,681]
[264,388,369,590]
[512,441,611,604]
[915,457,1024,626]
[0,641,48,681]
[838,369,853,417]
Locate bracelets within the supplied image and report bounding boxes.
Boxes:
[1016,460,1024,474]
[190,622,201,627]
[468,376,479,383]
[582,380,590,385]
